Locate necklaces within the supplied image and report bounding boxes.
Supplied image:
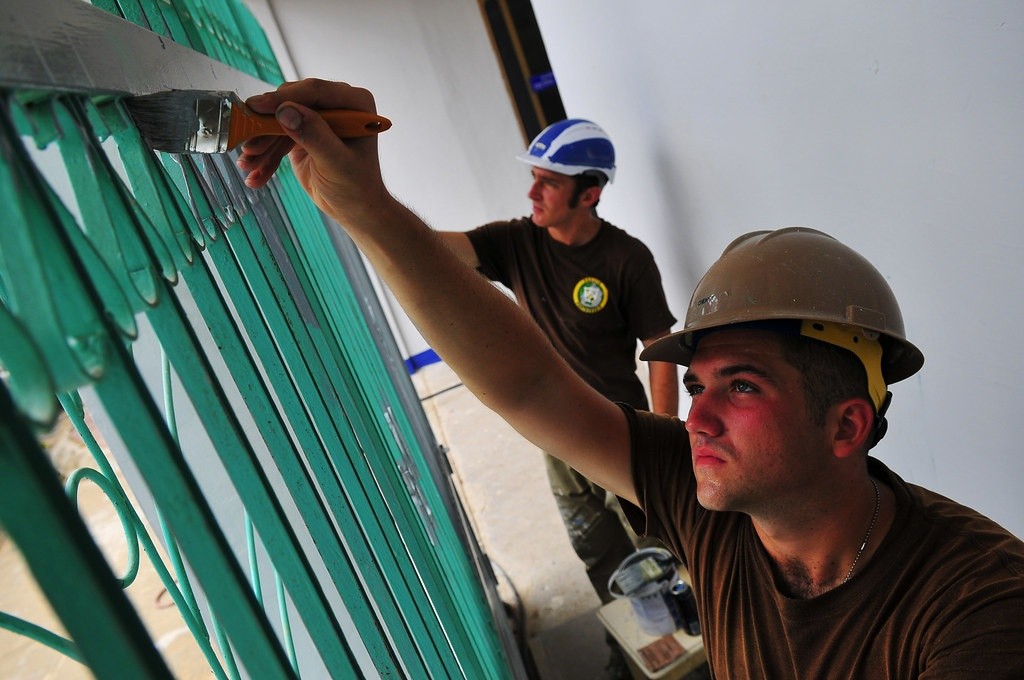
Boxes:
[835,477,884,580]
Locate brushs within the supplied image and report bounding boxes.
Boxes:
[125,88,393,154]
[614,554,679,596]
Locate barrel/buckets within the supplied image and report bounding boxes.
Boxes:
[608,548,679,637]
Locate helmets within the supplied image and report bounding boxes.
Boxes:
[514,119,616,183]
[639,227,925,385]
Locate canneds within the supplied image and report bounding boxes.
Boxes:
[670,582,701,636]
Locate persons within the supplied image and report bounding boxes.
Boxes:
[238,76,1024,680]
[426,119,676,680]
